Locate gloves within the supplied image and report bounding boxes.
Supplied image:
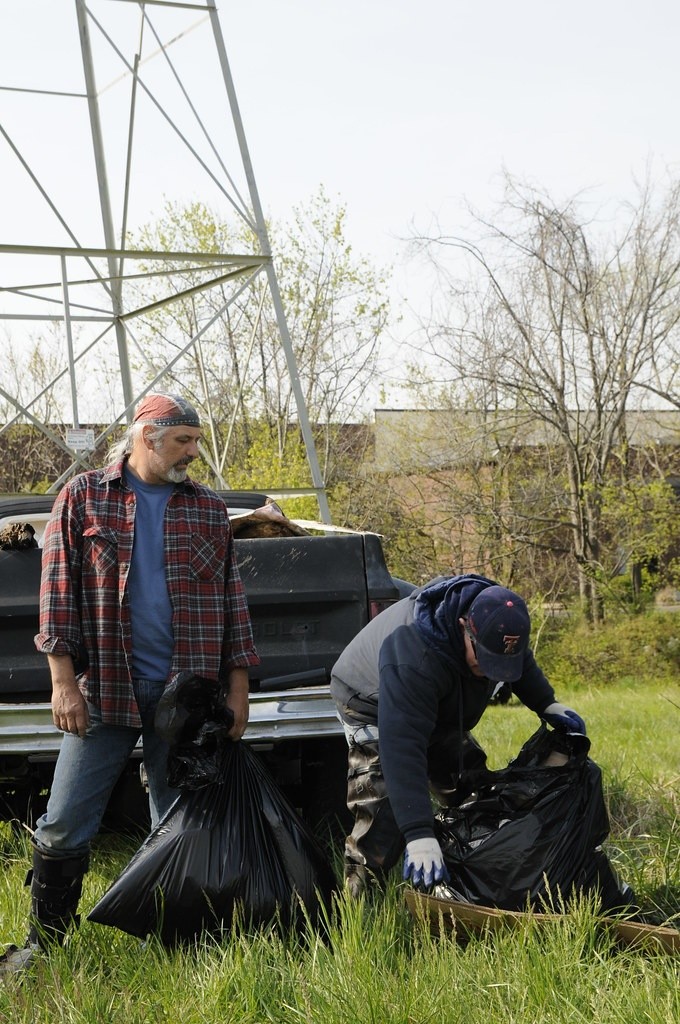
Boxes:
[403,828,450,888]
[544,703,586,736]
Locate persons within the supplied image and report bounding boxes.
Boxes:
[1,395,261,977]
[330,574,586,925]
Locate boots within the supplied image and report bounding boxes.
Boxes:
[0,833,91,986]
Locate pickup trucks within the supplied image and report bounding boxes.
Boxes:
[1,484,420,824]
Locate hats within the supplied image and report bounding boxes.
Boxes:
[468,585,531,682]
